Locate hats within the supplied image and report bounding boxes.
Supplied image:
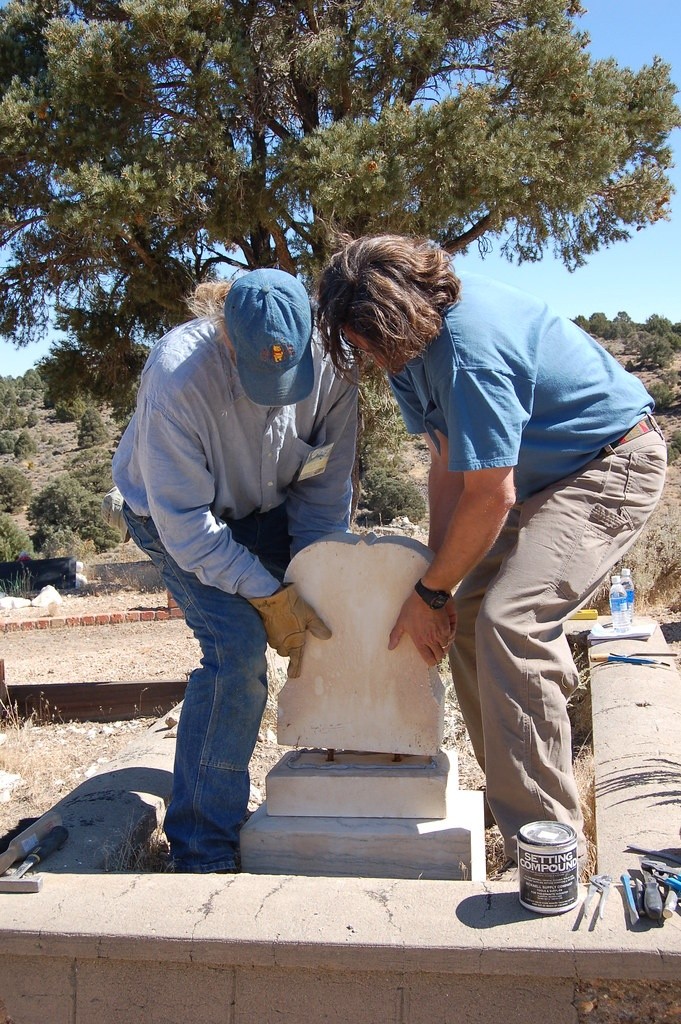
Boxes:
[225,269,314,407]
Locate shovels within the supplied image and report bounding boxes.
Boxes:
[639,858,665,919]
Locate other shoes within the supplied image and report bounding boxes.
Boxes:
[498,858,520,882]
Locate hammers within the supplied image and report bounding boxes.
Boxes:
[0,826,69,892]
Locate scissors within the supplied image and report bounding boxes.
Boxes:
[643,859,681,890]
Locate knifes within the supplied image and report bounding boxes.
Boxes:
[642,870,663,920]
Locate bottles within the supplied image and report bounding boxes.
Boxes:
[609,576,631,633]
[620,569,634,624]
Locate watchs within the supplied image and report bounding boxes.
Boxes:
[413,580,448,610]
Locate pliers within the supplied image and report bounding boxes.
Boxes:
[581,873,612,922]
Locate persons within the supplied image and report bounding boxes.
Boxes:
[113,268,358,873]
[317,232,679,878]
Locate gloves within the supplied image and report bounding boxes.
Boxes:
[247,582,332,679]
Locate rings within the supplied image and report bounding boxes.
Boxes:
[441,641,449,649]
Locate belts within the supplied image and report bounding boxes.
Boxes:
[593,414,656,457]
